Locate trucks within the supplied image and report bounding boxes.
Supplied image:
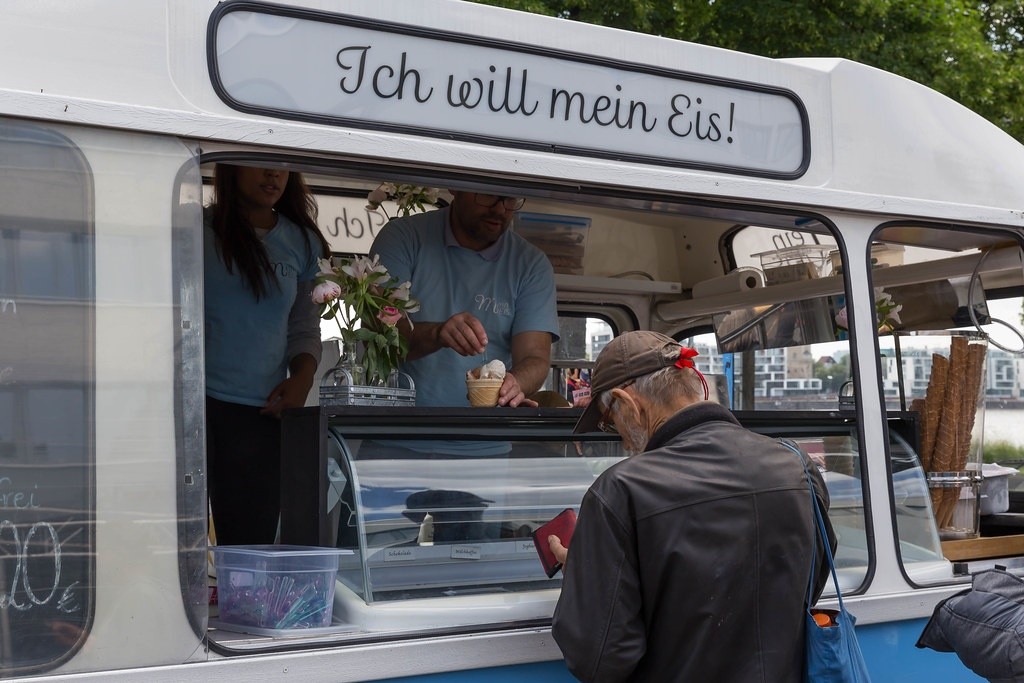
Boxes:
[1,0,1024,683]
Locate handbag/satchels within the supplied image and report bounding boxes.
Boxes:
[779,438,871,683]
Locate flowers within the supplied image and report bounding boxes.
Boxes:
[362,181,450,222]
[834,286,903,358]
[309,253,421,385]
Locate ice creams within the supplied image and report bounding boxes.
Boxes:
[465,359,506,407]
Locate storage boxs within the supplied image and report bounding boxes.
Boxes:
[206,544,356,637]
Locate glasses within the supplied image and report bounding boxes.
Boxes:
[474,193,526,211]
[596,379,635,434]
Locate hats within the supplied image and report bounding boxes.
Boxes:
[572,331,695,434]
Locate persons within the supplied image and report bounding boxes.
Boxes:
[563,364,591,456]
[914,569,1022,682]
[548,332,838,683]
[203,163,335,547]
[365,189,561,407]
